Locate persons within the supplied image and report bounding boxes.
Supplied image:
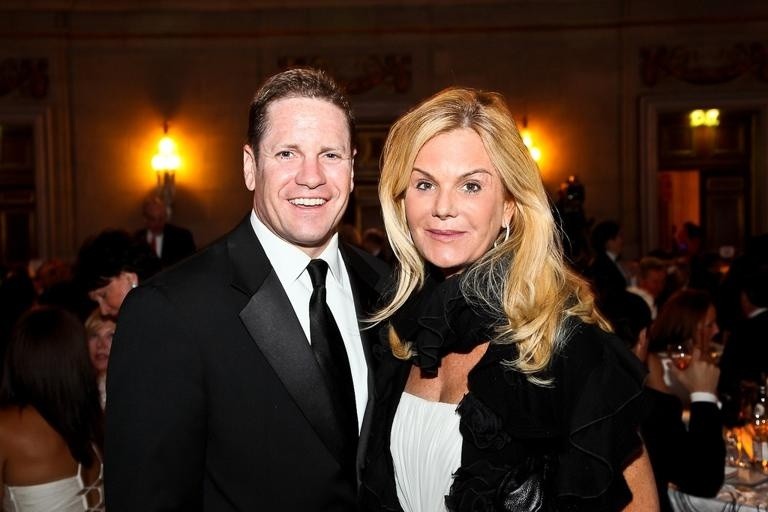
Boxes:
[551,178,768,511]
[102,65,395,512]
[350,86,660,512]
[1,195,195,512]
[342,226,399,270]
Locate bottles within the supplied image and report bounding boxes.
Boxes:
[751,385,767,473]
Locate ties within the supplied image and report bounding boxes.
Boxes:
[305,258,358,449]
[151,236,156,255]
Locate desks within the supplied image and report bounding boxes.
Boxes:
[668,424,768,512]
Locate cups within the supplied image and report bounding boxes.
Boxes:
[666,342,691,371]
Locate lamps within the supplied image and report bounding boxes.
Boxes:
[145,118,181,219]
